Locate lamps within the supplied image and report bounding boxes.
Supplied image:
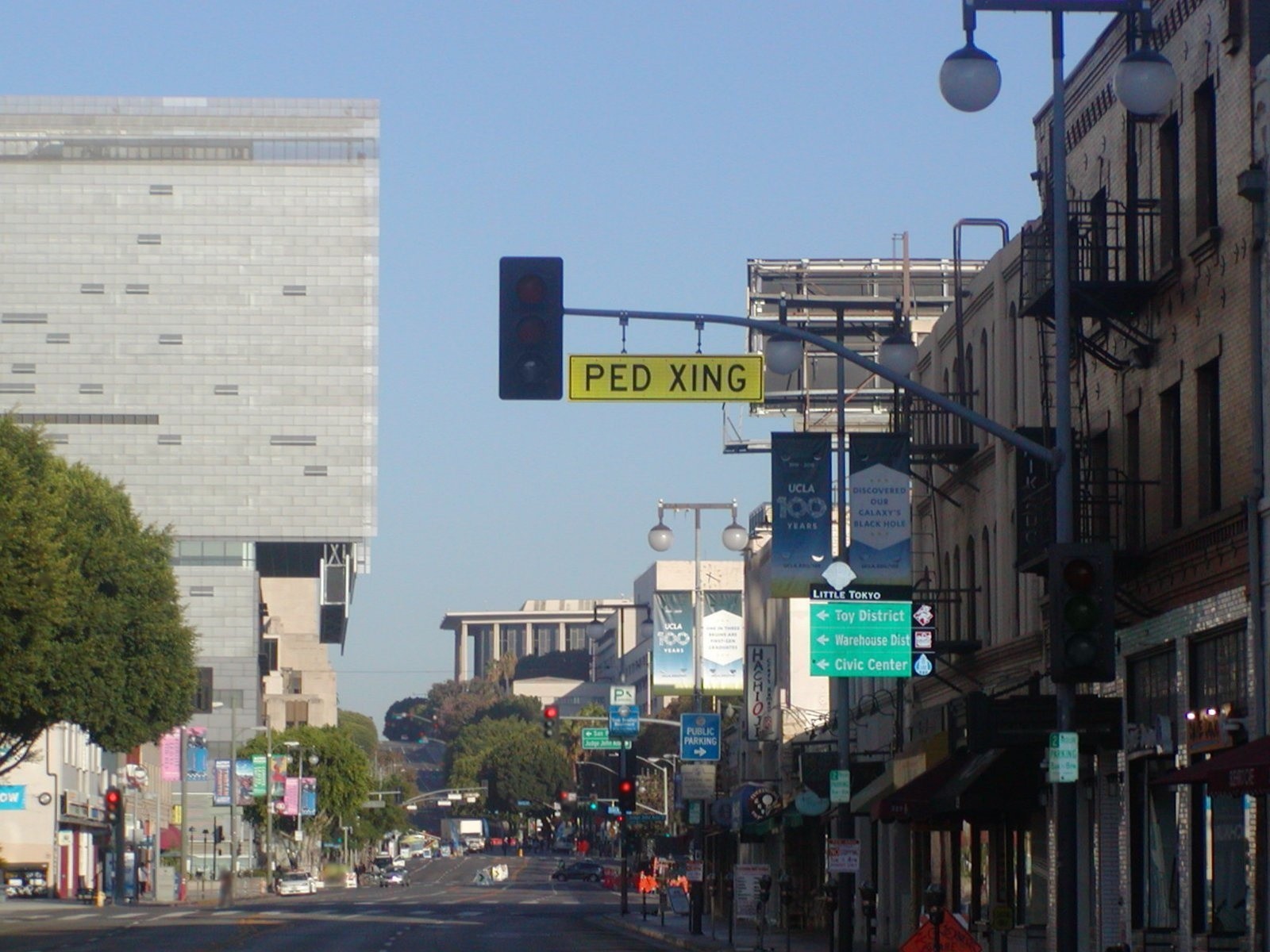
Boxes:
[1105,771,1125,797]
[1082,776,1097,801]
[810,689,898,741]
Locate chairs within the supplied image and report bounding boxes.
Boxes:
[76,875,93,905]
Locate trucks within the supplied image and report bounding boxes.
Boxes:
[440,818,486,853]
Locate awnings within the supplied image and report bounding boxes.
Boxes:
[3,863,49,872]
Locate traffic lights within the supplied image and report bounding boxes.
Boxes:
[105,789,124,824]
[501,260,566,400]
[544,704,560,740]
[1048,544,1121,684]
[590,793,599,812]
[618,776,638,813]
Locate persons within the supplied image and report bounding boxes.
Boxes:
[353,861,366,884]
[138,862,148,896]
[502,836,545,858]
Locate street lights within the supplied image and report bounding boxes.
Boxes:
[267,717,299,889]
[940,0,1180,951]
[229,697,269,898]
[587,601,655,910]
[179,701,226,881]
[649,756,680,823]
[747,294,926,951]
[648,500,747,933]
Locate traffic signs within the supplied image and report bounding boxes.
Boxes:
[681,713,721,762]
[583,728,633,751]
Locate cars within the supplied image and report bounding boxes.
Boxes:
[375,856,395,871]
[552,862,604,883]
[380,864,411,887]
[276,871,318,896]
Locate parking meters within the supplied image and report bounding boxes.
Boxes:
[724,873,737,941]
[758,873,875,951]
[926,883,944,951]
[710,873,719,939]
